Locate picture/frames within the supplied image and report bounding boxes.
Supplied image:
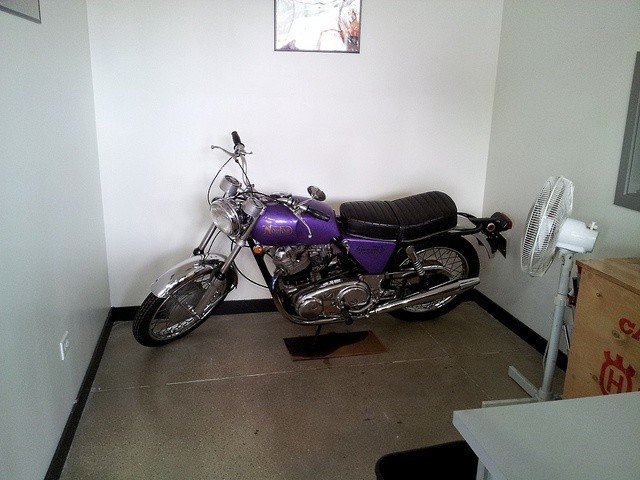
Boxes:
[0,0,42,24]
[614,51,640,213]
[273,0,363,53]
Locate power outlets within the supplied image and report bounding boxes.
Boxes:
[60,330,72,360]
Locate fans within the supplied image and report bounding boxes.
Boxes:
[482,174,599,408]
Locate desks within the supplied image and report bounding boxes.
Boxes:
[452,391,640,480]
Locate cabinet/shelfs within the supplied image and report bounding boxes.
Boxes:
[563,256,640,399]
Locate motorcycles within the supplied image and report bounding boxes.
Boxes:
[132,131,512,348]
